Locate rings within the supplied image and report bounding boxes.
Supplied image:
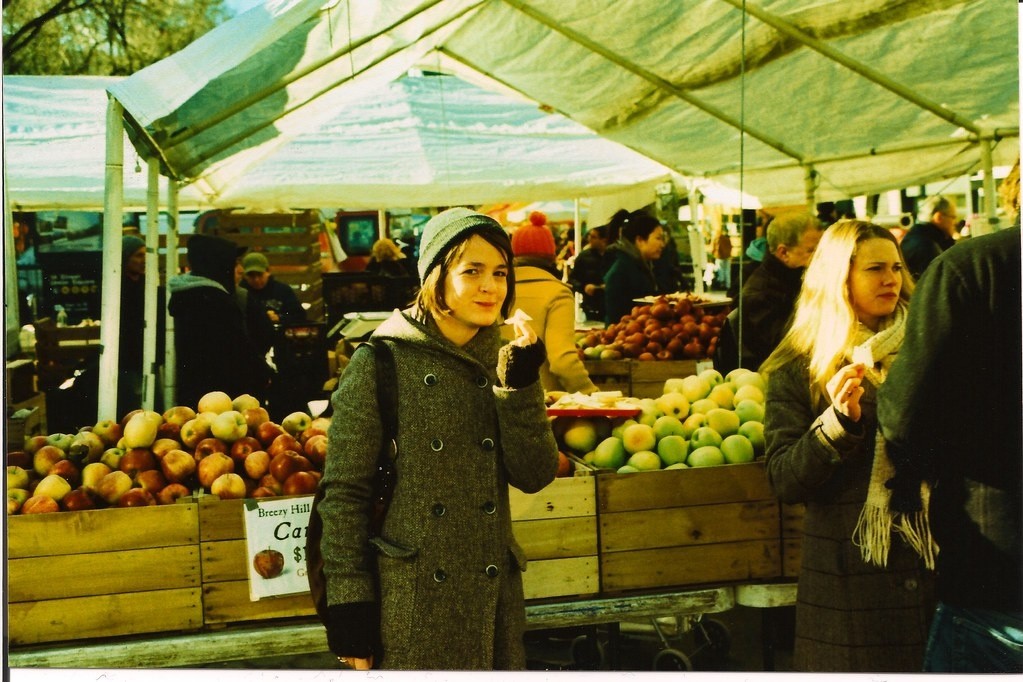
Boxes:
[338,656,349,663]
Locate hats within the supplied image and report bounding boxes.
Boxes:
[416,207,507,287]
[371,238,407,261]
[511,210,557,256]
[242,253,269,275]
[187,232,250,273]
[121,234,146,260]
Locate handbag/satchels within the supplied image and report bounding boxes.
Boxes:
[306,455,394,624]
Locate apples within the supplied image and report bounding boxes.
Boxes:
[8,296,766,513]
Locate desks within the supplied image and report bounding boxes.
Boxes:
[4,584,800,671]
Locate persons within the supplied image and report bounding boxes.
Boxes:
[100,207,1022,673]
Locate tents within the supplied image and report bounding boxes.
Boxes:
[3,1,1022,437]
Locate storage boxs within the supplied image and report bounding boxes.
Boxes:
[6,359,45,451]
[6,462,805,647]
[216,208,322,322]
[583,360,696,399]
